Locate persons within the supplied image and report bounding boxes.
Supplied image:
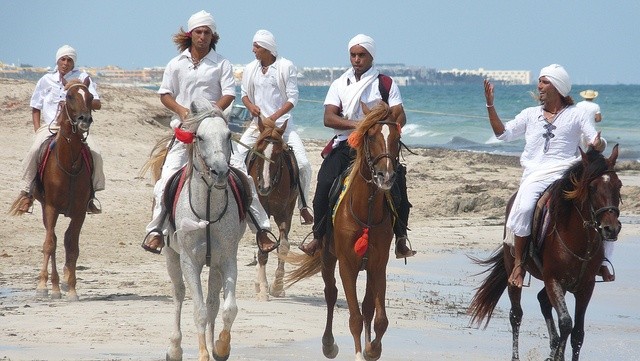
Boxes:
[13,44,105,214]
[232,29,313,223]
[483,64,607,288]
[306,33,416,253]
[146,11,277,252]
[576,89,602,134]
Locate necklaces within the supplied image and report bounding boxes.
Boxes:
[543,109,561,115]
[189,55,205,65]
[259,64,274,70]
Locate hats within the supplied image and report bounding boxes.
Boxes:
[580,90,598,99]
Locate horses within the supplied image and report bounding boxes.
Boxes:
[463,143,622,361]
[244,116,300,300]
[137,100,246,361]
[7,75,94,302]
[269,99,402,360]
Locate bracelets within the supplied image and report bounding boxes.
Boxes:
[486,103,496,108]
[598,140,604,149]
